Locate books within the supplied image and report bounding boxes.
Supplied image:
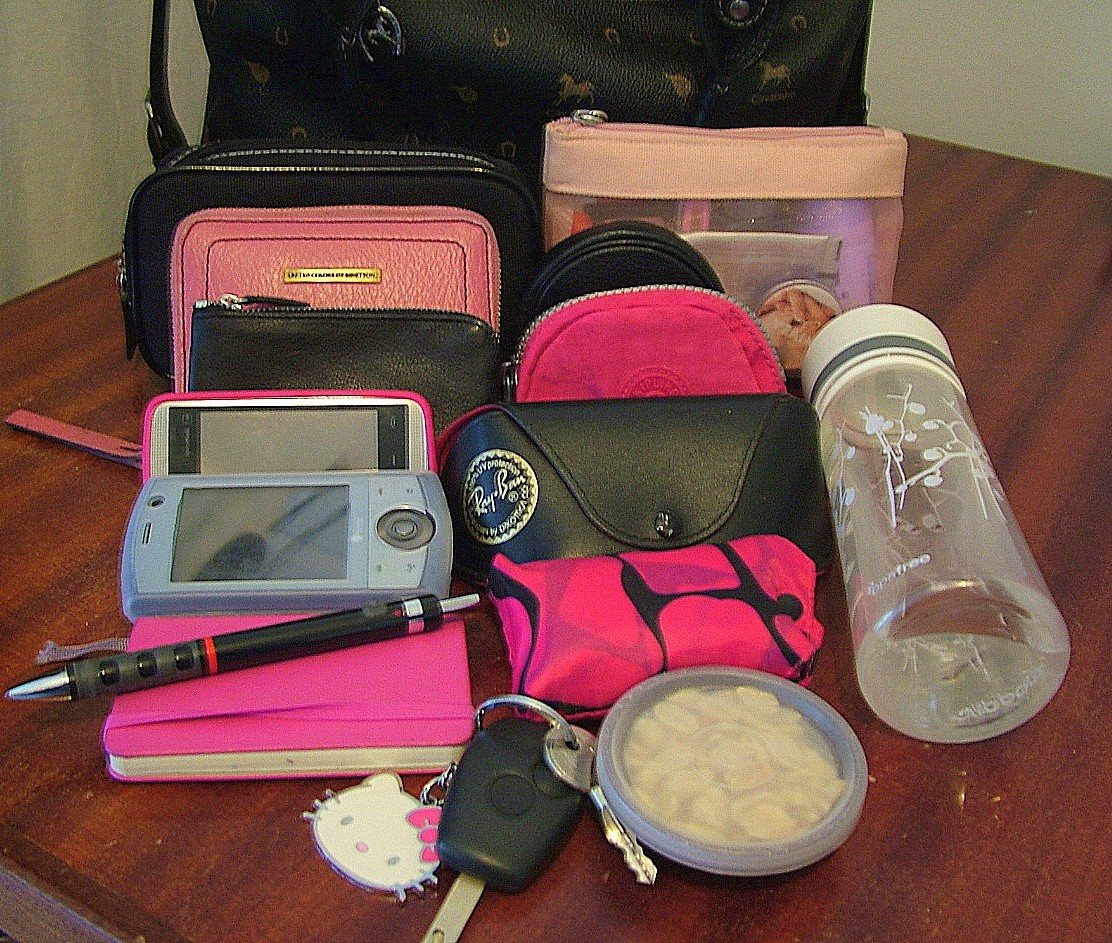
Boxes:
[99,609,475,781]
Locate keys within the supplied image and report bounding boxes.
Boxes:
[419,720,585,941]
[545,727,658,888]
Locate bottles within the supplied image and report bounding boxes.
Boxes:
[804,305,1069,744]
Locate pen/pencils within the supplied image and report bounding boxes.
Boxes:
[5,593,481,700]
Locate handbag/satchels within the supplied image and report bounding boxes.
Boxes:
[197,0,874,179]
[118,139,544,382]
[440,391,839,595]
[533,106,906,376]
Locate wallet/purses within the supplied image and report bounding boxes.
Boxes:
[164,203,506,392]
[522,219,722,332]
[485,544,822,721]
[508,285,792,396]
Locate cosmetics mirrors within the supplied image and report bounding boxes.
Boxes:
[753,279,841,379]
